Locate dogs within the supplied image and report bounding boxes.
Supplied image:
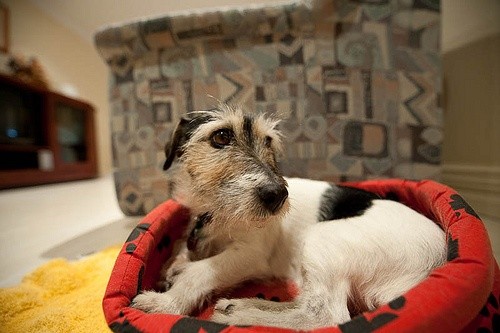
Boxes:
[127,93,449,333]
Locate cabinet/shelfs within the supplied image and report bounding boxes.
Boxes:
[0,72,99,187]
